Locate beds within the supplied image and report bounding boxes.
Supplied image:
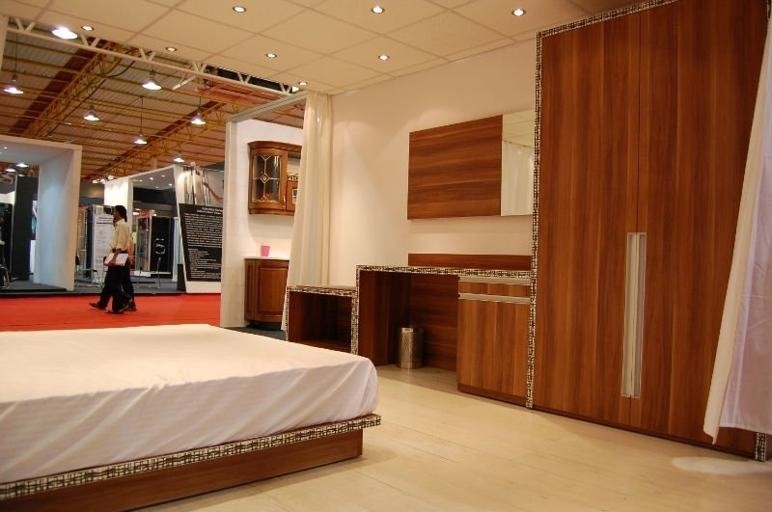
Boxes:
[1,322,386,512]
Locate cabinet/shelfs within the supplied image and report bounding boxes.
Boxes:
[246,138,303,215]
[243,257,293,330]
[285,251,536,407]
[530,0,770,470]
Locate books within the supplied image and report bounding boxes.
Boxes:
[102,253,129,268]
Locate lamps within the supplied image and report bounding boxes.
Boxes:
[1,30,26,96]
[190,91,210,128]
[133,93,152,146]
[142,61,163,93]
[83,63,102,120]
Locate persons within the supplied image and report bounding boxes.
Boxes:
[107,204,131,313]
[88,217,136,311]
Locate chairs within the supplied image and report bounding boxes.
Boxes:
[73,253,105,291]
[137,254,164,289]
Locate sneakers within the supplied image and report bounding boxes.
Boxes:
[109,304,136,314]
[90,303,106,311]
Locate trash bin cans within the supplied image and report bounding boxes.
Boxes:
[398,326,425,368]
